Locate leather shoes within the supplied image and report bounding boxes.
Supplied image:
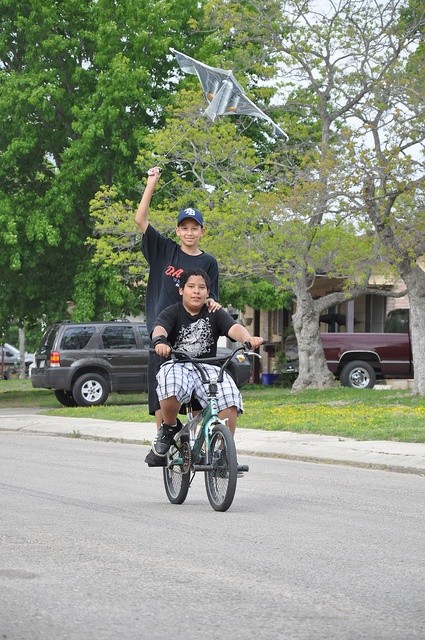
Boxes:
[145,449,167,466]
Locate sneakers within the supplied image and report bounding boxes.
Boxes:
[151,418,184,457]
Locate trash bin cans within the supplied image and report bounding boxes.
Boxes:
[261,374,279,385]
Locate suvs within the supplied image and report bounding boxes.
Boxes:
[32,322,250,406]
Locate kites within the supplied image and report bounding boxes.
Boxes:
[168,47,292,143]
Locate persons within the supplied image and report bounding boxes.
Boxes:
[132,167,244,479]
[149,268,264,473]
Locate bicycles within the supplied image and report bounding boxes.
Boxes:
[148,339,267,511]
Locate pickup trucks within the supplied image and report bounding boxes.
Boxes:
[280,309,414,389]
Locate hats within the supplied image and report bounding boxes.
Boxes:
[178,209,202,226]
